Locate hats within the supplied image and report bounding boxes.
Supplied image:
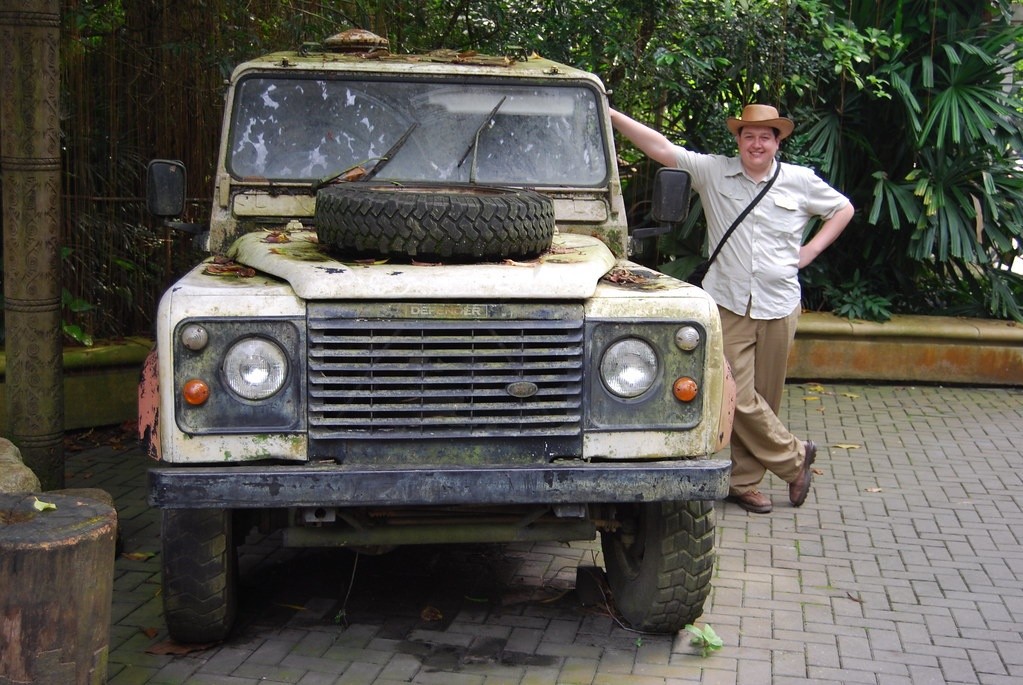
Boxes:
[726,104,794,142]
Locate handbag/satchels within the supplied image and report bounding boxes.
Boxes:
[685,261,710,289]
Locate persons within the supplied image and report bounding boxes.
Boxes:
[608,104,854,513]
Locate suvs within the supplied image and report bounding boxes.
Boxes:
[136,30,738,644]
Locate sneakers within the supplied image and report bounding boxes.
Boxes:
[788,439,818,507]
[725,488,772,514]
